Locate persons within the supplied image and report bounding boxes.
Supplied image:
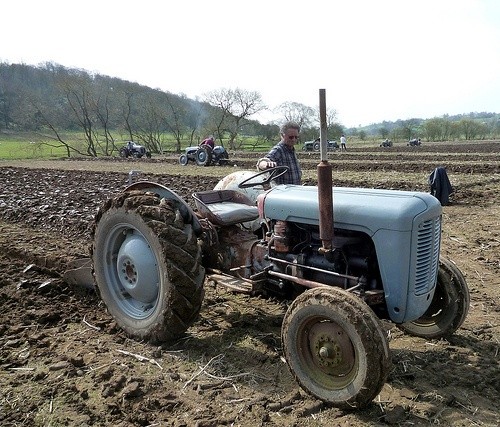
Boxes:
[255,120,303,186]
[198,135,215,149]
[127,142,134,152]
[340,135,348,152]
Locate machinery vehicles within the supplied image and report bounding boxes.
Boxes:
[87,89,472,410]
[118,141,151,159]
[178,143,229,166]
[407,138,421,146]
[380,139,393,147]
[302,137,338,152]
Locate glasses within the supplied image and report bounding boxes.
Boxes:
[286,135,298,140]
